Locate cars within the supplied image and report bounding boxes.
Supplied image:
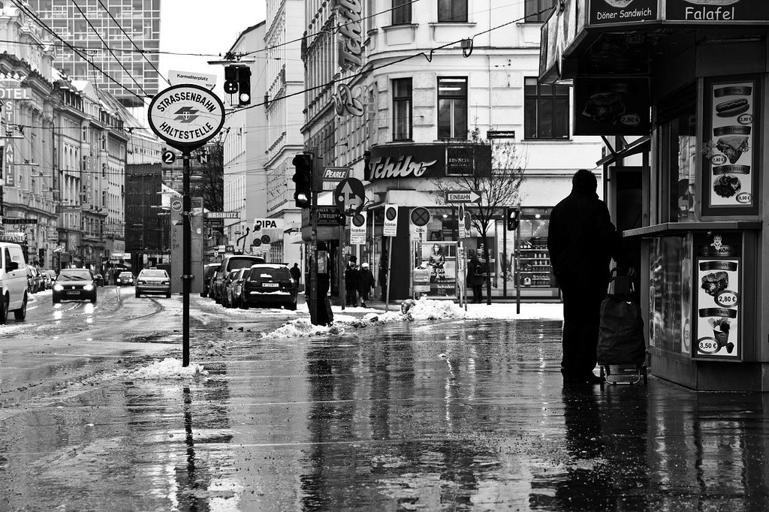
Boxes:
[26,255,298,316]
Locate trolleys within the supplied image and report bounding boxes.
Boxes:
[594,263,652,391]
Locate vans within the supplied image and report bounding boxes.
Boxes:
[0,241,30,327]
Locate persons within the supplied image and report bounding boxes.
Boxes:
[547,169,636,387]
[430,244,446,279]
[289,263,301,292]
[467,253,485,303]
[344,255,375,308]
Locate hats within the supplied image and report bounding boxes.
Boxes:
[362,263,369,268]
[348,255,357,261]
[350,262,355,267]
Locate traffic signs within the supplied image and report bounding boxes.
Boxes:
[442,189,482,203]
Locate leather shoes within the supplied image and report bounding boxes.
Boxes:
[561,367,607,387]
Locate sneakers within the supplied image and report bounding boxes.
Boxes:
[346,301,367,308]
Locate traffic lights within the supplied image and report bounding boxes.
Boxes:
[508,208,520,230]
[237,65,252,106]
[223,66,239,94]
[290,154,313,209]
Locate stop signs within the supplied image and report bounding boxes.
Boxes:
[386,207,397,221]
[352,213,365,227]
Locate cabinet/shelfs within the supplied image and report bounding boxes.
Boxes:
[514,247,552,288]
